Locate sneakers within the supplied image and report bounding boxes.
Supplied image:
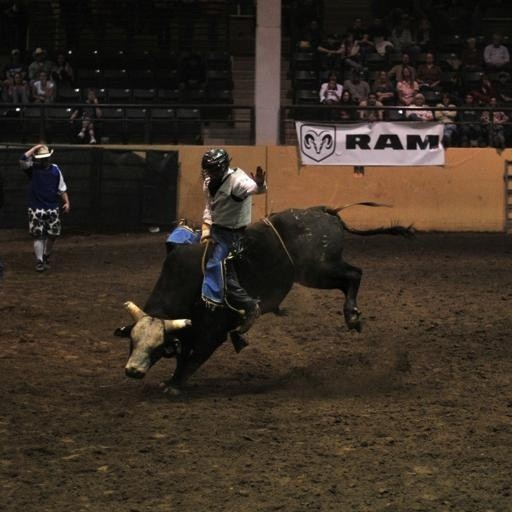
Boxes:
[34,253,50,271]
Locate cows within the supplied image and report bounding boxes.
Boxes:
[112,201,396,398]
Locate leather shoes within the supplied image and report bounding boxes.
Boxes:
[238,303,261,335]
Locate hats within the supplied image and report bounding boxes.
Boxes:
[32,145,54,158]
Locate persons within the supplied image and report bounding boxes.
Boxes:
[19,144,71,272]
[317,5,510,147]
[196,148,267,334]
[1,6,104,143]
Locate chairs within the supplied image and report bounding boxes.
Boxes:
[0,39,236,148]
[288,11,511,121]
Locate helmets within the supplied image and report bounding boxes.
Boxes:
[201,147,230,186]
